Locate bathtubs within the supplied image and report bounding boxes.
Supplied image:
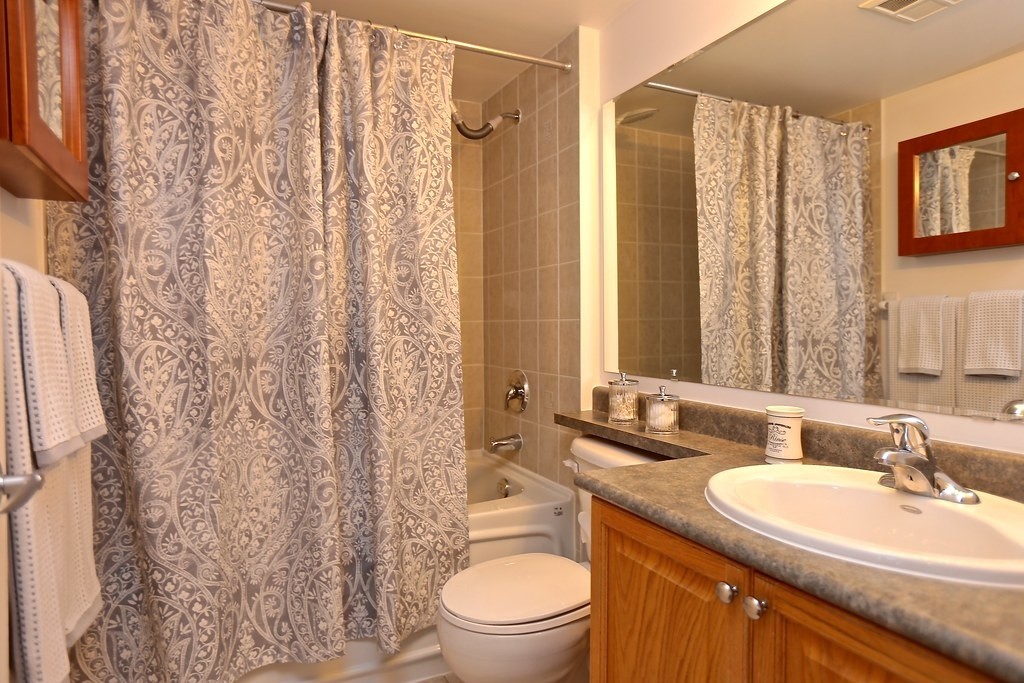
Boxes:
[234,454,575,683]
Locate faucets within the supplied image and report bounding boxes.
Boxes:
[1001,400,1023,419]
[867,413,980,504]
[488,433,523,453]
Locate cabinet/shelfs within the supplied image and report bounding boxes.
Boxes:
[589,500,992,683]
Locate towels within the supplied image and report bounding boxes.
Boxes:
[0,256,107,683]
[886,290,1024,416]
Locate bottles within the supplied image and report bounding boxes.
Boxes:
[645,386,680,435]
[607,372,640,425]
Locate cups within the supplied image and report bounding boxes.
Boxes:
[764,405,805,465]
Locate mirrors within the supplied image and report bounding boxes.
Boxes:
[8,0,90,197]
[898,108,1024,257]
[603,2,1024,422]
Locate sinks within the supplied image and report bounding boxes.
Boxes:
[703,463,1024,588]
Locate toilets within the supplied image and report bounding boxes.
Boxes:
[436,434,656,683]
